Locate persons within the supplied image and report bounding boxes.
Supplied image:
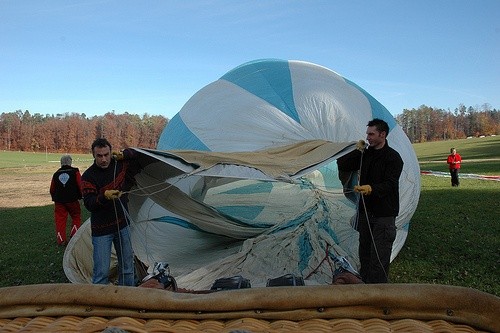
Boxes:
[354,118,404,283]
[80,137,134,287]
[447,147,462,187]
[49,155,82,245]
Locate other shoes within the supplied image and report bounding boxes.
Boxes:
[58,240,68,247]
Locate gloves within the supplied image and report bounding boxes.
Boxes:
[354,184,372,196]
[104,190,123,199]
[356,139,369,153]
[112,150,123,160]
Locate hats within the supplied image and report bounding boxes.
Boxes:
[61,154,72,165]
